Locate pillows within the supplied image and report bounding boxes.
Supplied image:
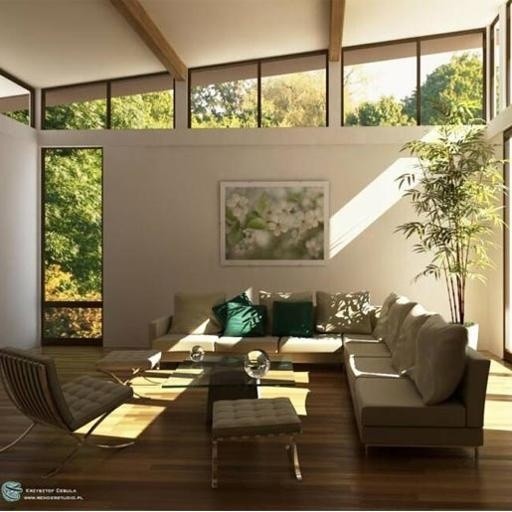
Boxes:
[256,288,316,332]
[270,297,315,340]
[171,289,226,337]
[314,289,375,335]
[389,303,437,370]
[222,299,269,340]
[369,290,419,353]
[210,292,254,338]
[403,312,470,406]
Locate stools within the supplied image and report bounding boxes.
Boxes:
[205,395,306,490]
[93,347,164,406]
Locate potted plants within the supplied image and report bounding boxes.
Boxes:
[391,88,511,356]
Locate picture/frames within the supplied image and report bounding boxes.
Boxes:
[218,180,331,268]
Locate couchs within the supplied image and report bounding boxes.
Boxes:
[147,284,492,460]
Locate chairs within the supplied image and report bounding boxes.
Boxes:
[1,344,140,481]
